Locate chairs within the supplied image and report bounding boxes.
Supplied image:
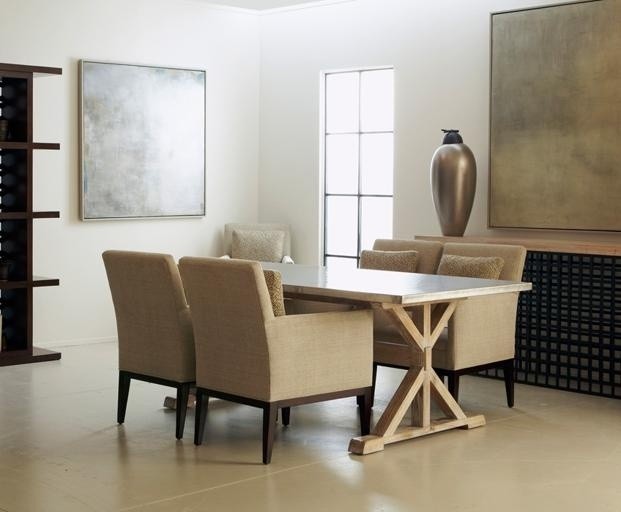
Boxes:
[371,242,527,408]
[177,255,374,464]
[219,223,295,265]
[356,238,442,406]
[102,250,278,442]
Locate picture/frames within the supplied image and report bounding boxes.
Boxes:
[489,2,621,233]
[77,58,208,222]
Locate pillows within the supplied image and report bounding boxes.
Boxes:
[359,249,419,273]
[231,230,285,263]
[264,270,285,318]
[437,254,505,280]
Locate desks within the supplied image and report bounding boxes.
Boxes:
[258,259,533,455]
